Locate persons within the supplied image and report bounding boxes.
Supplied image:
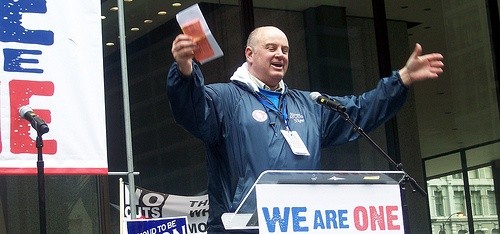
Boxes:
[166,25,445,234]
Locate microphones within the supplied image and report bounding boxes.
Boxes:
[308,92,346,112]
[19,106,50,134]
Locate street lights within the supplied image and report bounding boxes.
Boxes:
[442,212,463,234]
